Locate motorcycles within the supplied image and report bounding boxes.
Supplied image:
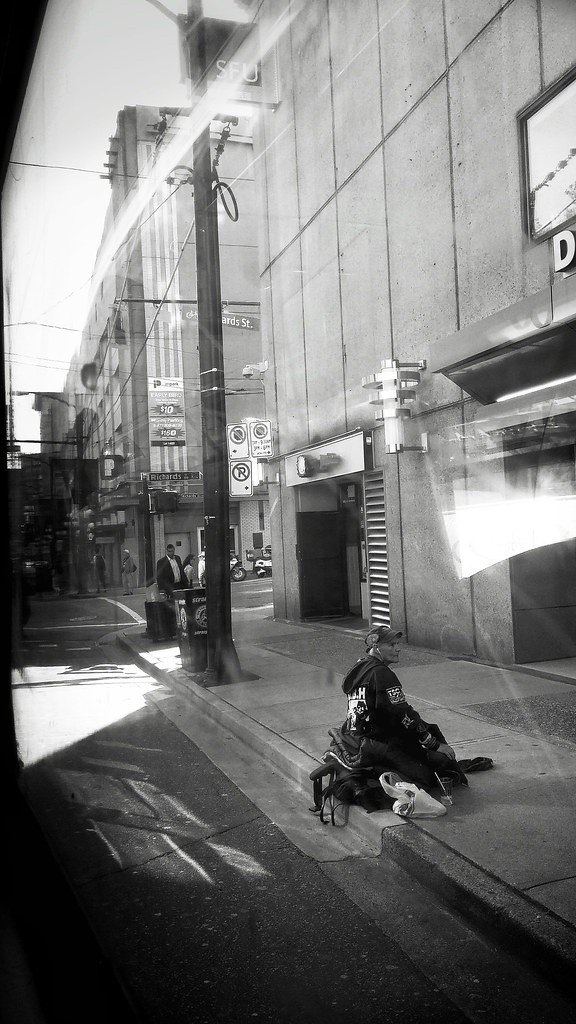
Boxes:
[229,553,246,581]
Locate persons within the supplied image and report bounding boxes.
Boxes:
[184,562,193,587]
[343,626,460,788]
[95,553,106,593]
[31,564,43,598]
[122,550,133,595]
[198,555,205,587]
[158,544,189,597]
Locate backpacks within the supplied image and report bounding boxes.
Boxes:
[94,554,105,570]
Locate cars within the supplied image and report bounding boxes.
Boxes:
[252,545,272,578]
[22,553,49,574]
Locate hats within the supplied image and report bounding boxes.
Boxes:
[364,627,403,654]
[198,551,206,558]
[123,549,130,554]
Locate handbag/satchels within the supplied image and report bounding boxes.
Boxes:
[379,770,447,820]
[130,563,136,572]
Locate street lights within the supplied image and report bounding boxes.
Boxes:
[17,391,90,594]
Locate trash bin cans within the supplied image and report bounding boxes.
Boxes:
[174,586,208,673]
[146,588,176,643]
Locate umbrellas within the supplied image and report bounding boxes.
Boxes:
[183,554,194,565]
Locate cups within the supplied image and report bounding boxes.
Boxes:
[437,777,454,806]
